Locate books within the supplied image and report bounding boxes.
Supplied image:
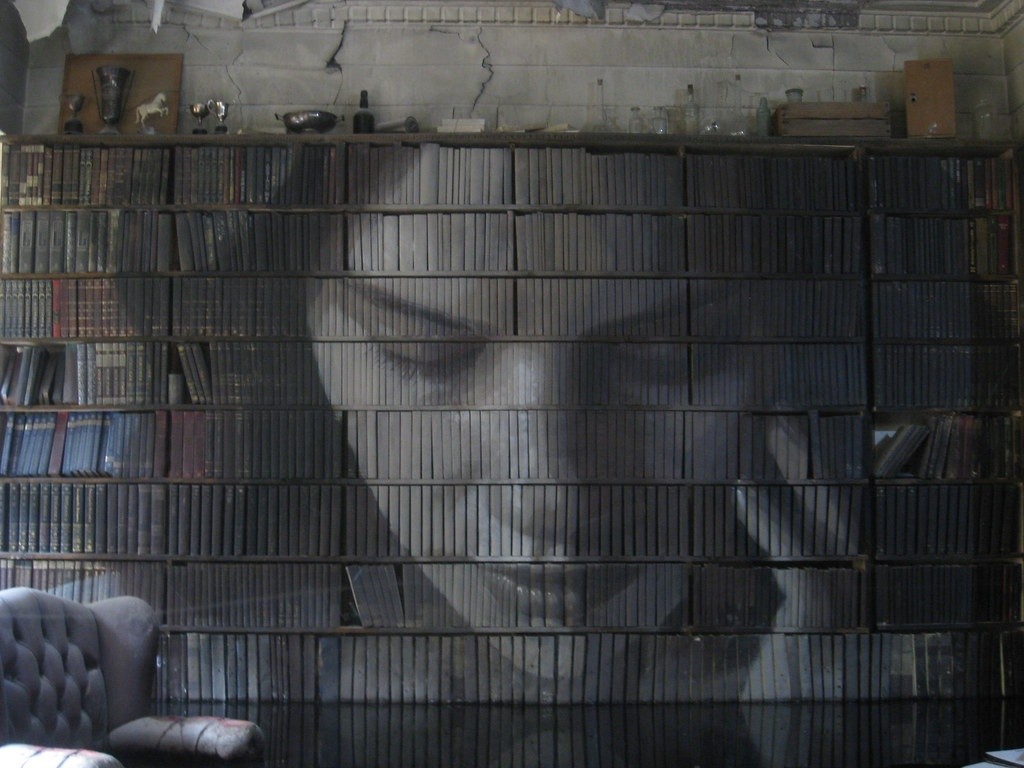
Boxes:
[2,143,1024,767]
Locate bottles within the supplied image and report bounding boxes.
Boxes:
[727,74,752,136]
[588,78,608,131]
[756,97,771,136]
[858,85,866,103]
[651,106,668,134]
[629,107,644,133]
[974,99,999,140]
[683,84,699,134]
[353,90,375,134]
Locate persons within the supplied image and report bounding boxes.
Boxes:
[93,142,918,768]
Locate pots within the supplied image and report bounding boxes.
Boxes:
[275,111,346,134]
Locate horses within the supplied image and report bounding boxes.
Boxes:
[134,92,169,129]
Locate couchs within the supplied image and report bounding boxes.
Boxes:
[0,586,268,768]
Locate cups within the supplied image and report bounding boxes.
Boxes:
[785,89,803,103]
[168,373,186,403]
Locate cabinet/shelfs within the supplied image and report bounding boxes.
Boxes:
[0,131,1024,708]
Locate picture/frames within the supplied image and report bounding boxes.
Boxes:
[57,53,184,133]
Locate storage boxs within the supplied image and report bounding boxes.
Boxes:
[904,59,956,139]
[776,101,893,137]
